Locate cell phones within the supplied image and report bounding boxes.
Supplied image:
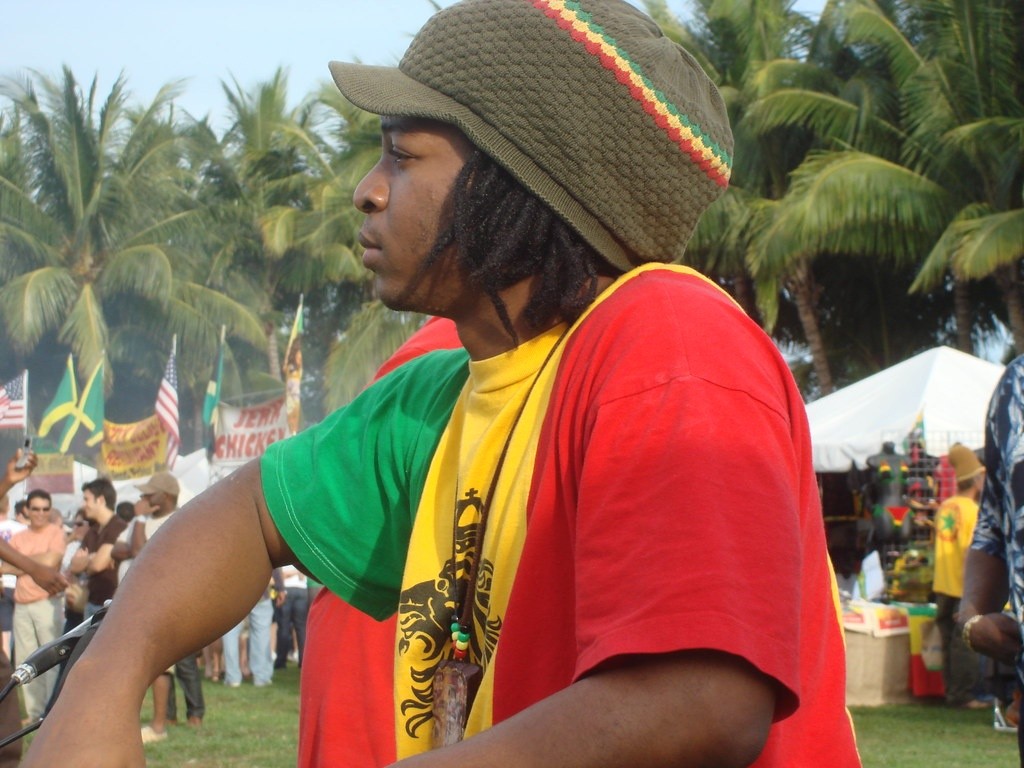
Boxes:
[13,436,33,468]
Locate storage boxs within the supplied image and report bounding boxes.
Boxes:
[845,622,912,706]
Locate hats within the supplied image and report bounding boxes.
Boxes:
[949,443,987,483]
[328,0,735,272]
[133,472,180,498]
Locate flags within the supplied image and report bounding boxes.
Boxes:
[39,352,103,447]
[153,336,183,467]
[281,298,308,432]
[203,322,225,451]
[0,361,30,433]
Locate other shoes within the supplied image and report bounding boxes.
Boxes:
[962,697,992,712]
[187,716,201,726]
[274,658,285,668]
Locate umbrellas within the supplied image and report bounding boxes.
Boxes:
[803,341,1008,472]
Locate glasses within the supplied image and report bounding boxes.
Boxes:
[28,506,51,512]
[75,522,87,526]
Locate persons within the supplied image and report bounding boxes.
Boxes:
[931,353,1024,768]
[17,0,866,768]
[1,446,311,767]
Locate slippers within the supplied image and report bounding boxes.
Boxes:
[140,727,166,744]
